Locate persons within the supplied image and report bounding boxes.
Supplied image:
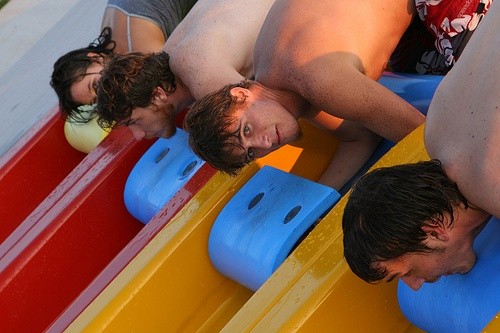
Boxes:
[49,0,198,124]
[341,0,500,290]
[94,0,276,142]
[185,0,494,190]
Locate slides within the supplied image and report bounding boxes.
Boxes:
[0,97,500,332]
